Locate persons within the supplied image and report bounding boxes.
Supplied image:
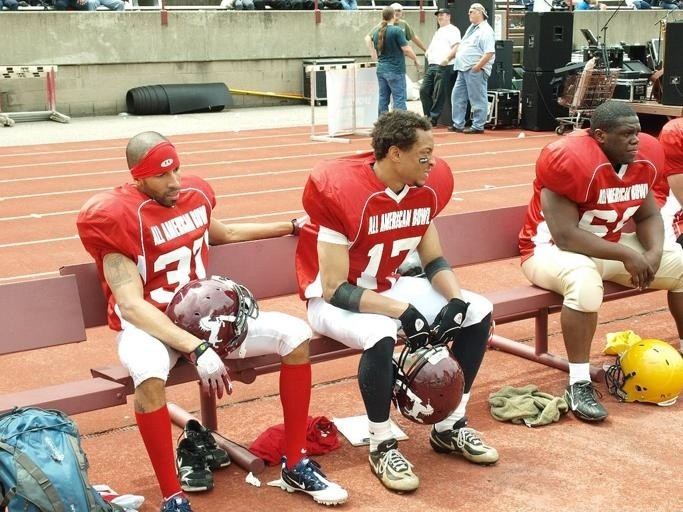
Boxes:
[519,101,683,423]
[296,111,498,492]
[0,1,141,11]
[366,3,426,101]
[419,8,462,127]
[519,0,683,10]
[374,6,419,117]
[659,109,683,245]
[76,131,349,512]
[448,3,496,134]
[221,0,358,10]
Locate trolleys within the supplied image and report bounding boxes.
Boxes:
[554,64,622,137]
[470,89,522,130]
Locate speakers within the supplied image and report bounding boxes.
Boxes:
[662,23,683,106]
[487,40,513,90]
[521,72,573,132]
[438,0,494,40]
[523,12,574,72]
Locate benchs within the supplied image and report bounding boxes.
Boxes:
[60,205,663,474]
[0,273,127,415]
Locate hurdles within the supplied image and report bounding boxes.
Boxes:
[305,60,420,144]
[0,65,71,125]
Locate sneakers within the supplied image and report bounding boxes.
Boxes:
[183,419,231,471]
[368,435,421,495]
[159,492,192,512]
[425,119,485,135]
[174,437,216,493]
[563,375,610,422]
[430,417,500,468]
[280,454,348,507]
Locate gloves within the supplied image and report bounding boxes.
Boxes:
[429,297,469,348]
[398,303,430,355]
[187,341,234,402]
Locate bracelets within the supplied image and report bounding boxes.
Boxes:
[292,218,299,234]
[329,281,364,311]
[423,258,456,286]
[191,340,210,366]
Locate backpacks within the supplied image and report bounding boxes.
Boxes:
[0,404,116,512]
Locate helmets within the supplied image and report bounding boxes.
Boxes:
[603,338,683,407]
[388,328,466,427]
[165,273,260,366]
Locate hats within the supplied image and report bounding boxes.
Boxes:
[434,7,451,15]
[468,3,490,18]
[388,3,405,12]
